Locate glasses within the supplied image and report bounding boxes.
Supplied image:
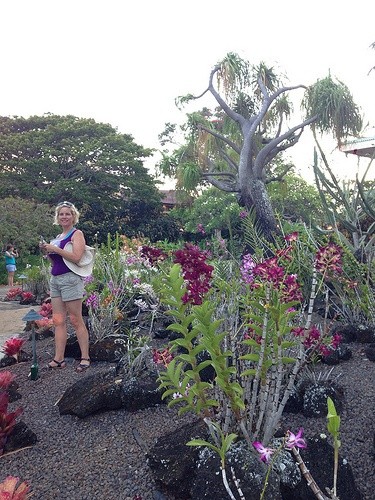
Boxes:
[58,202,72,206]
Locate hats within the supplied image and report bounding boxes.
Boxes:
[62,241,95,277]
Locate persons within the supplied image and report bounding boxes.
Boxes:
[39,201,91,372]
[5,245,19,288]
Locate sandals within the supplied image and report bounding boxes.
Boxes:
[45,360,65,369]
[76,358,91,371]
